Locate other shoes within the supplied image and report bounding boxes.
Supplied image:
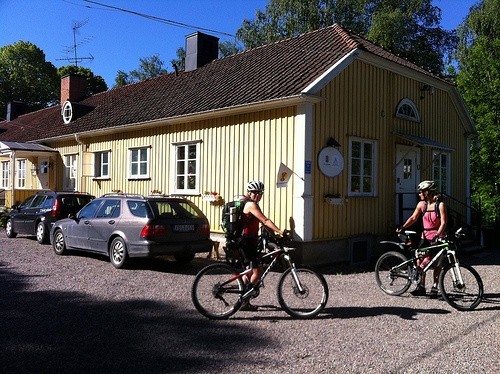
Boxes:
[238,302,259,311]
[410,285,426,296]
[429,287,438,298]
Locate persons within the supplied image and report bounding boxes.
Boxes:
[222,181,293,311]
[396,181,446,297]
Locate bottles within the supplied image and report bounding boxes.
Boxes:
[420,257,430,269]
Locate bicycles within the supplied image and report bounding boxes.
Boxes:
[374,226,484,312]
[190,229,330,321]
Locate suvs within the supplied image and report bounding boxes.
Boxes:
[48,193,213,270]
[4,189,97,245]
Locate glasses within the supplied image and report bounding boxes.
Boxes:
[249,190,264,194]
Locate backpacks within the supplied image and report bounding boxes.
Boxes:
[222,199,255,239]
[423,202,451,231]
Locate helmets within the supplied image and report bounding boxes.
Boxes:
[417,181,437,190]
[247,181,264,190]
